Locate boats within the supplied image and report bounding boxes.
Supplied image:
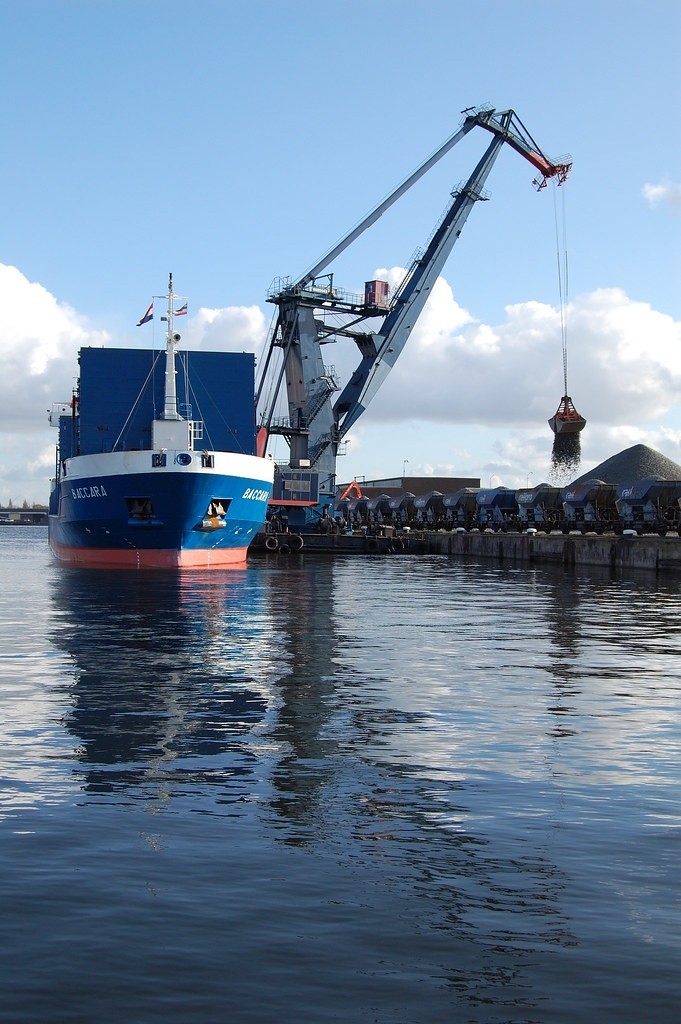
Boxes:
[46,273,276,571]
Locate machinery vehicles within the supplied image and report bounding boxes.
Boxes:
[255,99,586,503]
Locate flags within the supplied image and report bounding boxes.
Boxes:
[175,303,187,316]
[136,303,153,326]
